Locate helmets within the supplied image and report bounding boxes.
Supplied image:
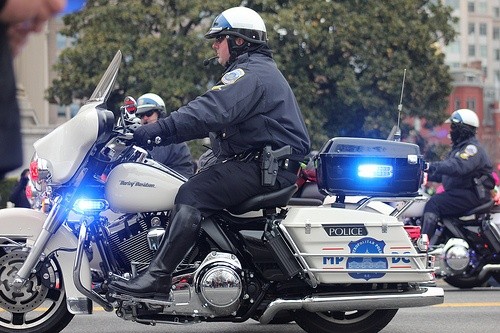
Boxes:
[444,108,480,128]
[136,93,166,114]
[204,6,268,43]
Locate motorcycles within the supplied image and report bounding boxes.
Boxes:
[386,69,500,288]
[0,49,445,333]
[316,194,403,222]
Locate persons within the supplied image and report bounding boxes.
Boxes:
[415,108,495,254]
[135,93,194,179]
[0,0,68,208]
[107,7,312,300]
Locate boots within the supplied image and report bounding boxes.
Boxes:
[420,211,438,241]
[108,204,203,301]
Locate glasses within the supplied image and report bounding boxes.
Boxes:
[214,35,231,43]
[138,111,156,118]
